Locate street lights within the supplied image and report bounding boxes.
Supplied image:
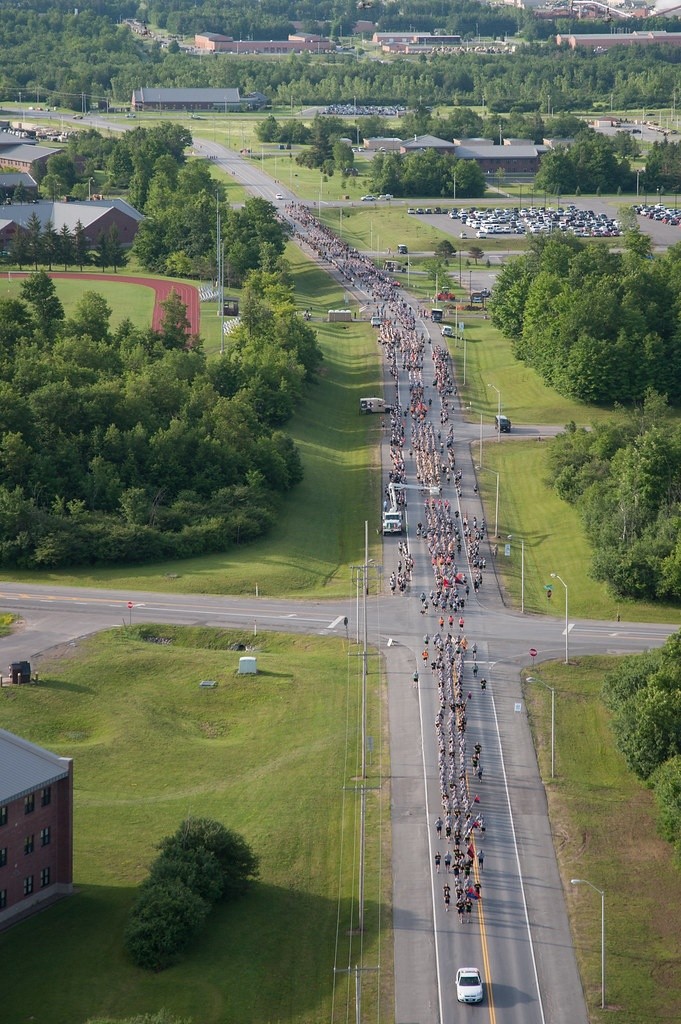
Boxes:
[473,466,500,537]
[526,677,556,779]
[487,383,501,443]
[570,879,605,1008]
[507,534,524,614]
[550,573,570,665]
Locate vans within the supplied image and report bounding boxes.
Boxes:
[431,308,443,323]
[494,414,510,432]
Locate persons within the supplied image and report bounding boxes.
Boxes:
[279,186,511,928]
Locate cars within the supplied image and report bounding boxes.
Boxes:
[406,201,681,240]
[454,967,483,1004]
[398,244,408,255]
[361,195,376,201]
[436,292,455,301]
[471,292,483,303]
[441,325,452,337]
[371,316,381,328]
[276,193,283,200]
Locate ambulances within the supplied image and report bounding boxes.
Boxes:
[359,398,393,415]
[384,260,406,273]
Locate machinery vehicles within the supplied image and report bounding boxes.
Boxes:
[382,483,441,536]
